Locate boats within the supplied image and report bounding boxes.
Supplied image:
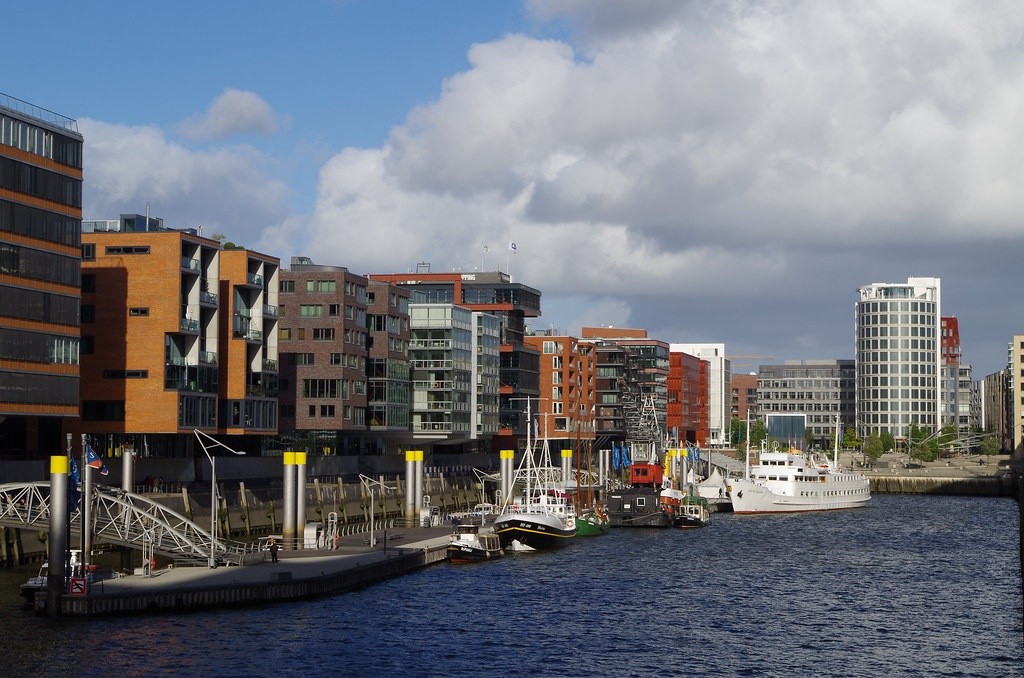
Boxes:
[599,376,733,528]
[724,408,872,513]
[474,503,495,512]
[20,550,82,605]
[446,524,505,563]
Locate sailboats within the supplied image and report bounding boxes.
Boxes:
[494,396,579,551]
[575,429,610,537]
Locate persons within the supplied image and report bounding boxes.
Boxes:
[718,488,723,498]
[142,474,182,494]
[451,515,461,525]
[514,503,519,515]
[269,543,279,562]
[1,494,36,519]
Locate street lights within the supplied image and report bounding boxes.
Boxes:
[359,474,398,548]
[194,429,246,568]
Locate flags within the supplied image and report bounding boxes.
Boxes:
[67,454,79,512]
[86,444,109,475]
[612,447,631,471]
